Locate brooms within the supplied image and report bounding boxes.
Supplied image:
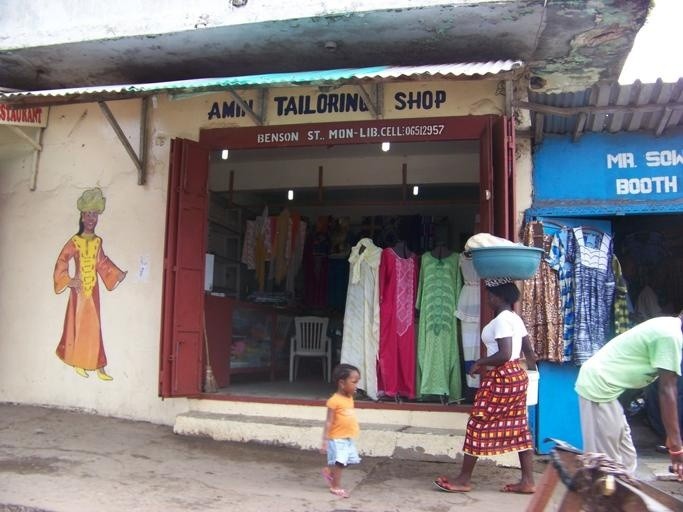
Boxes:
[203,311,222,394]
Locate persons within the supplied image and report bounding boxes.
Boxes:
[438,283,536,493]
[620,251,683,439]
[319,364,361,498]
[53,187,128,380]
[574,311,683,482]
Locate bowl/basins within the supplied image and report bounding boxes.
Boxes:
[468,247,544,281]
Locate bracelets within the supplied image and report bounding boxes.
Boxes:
[668,446,683,456]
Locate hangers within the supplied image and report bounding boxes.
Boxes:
[528,217,609,239]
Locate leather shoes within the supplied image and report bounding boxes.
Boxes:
[77,367,88,378]
[96,369,112,380]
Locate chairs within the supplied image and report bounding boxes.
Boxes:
[289,314,332,383]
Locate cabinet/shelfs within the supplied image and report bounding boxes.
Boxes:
[204,293,276,387]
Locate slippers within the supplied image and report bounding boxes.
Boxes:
[433,476,470,494]
[323,467,351,498]
[500,483,537,495]
